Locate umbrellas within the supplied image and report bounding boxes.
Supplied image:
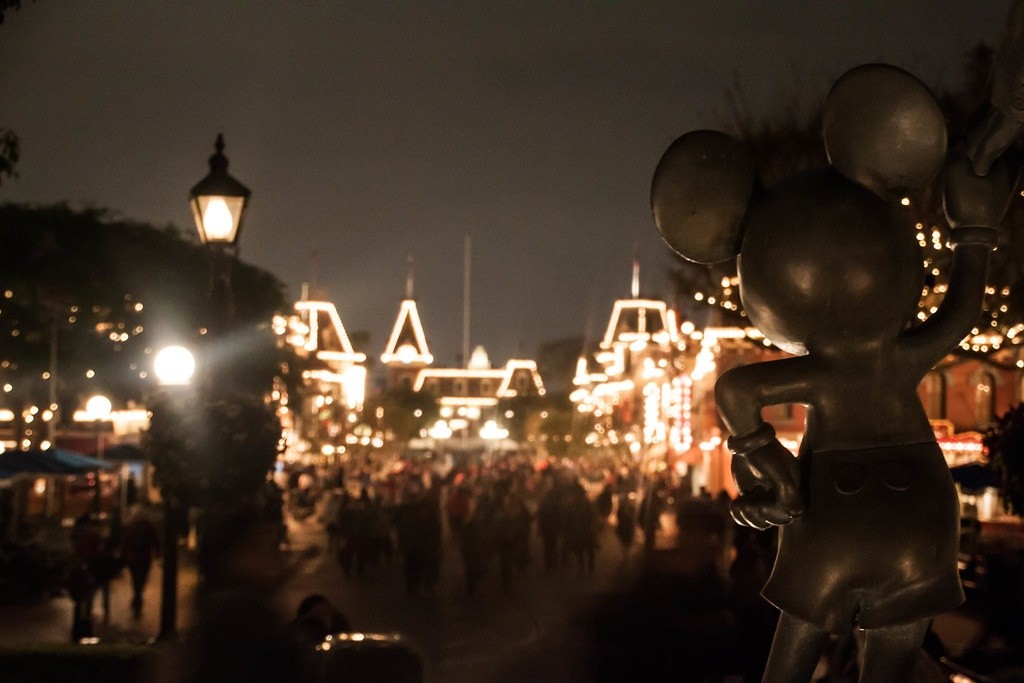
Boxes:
[1,448,106,474]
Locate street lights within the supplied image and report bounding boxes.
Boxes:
[151,345,197,646]
[186,130,254,366]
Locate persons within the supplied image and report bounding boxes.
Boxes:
[0,449,779,640]
